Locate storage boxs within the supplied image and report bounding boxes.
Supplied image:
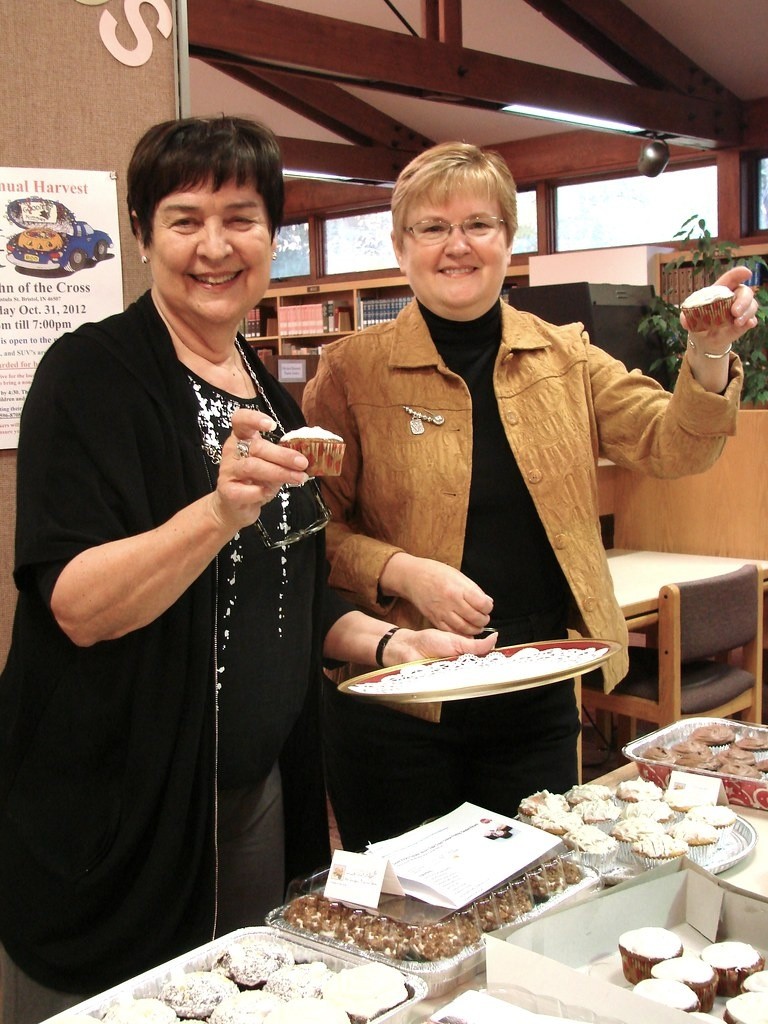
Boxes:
[485,855,768,1024]
[621,716,768,811]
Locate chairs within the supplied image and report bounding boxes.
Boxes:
[584,563,765,764]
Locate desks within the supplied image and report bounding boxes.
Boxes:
[604,546,768,631]
[588,760,768,896]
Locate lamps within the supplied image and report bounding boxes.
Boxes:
[637,132,671,179]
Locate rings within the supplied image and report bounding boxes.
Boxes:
[236,439,250,458]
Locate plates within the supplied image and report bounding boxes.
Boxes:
[337,639,623,703]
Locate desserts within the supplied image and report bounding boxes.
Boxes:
[279,426,346,477]
[618,926,768,1024]
[38,933,410,1024]
[680,285,735,333]
[517,774,737,875]
[640,724,768,781]
[283,853,582,966]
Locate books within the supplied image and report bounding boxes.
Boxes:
[237,296,413,364]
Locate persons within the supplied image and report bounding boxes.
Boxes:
[298,142,759,851]
[0,117,335,1024]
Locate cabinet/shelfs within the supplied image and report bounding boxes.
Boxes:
[238,263,531,355]
[655,242,768,298]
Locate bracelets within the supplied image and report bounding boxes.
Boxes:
[687,333,733,359]
[376,627,401,668]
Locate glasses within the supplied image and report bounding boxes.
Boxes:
[255,479,333,552]
[403,216,506,245]
